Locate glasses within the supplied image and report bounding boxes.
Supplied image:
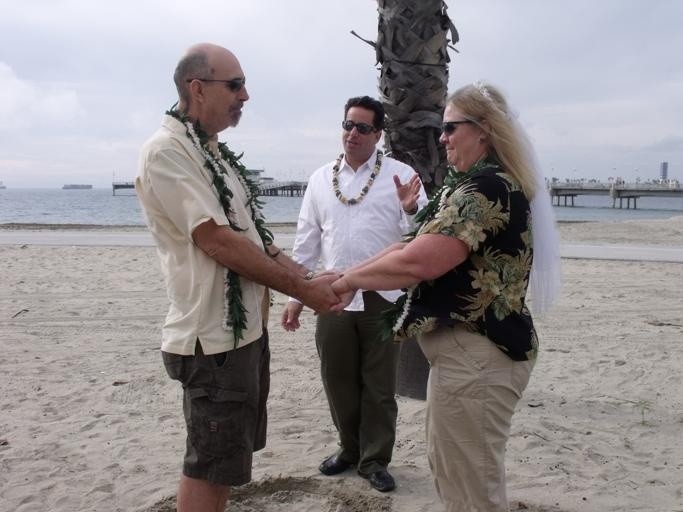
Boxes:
[342,121,378,135]
[186,77,245,93]
[441,120,472,133]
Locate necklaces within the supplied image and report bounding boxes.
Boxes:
[332,150,382,204]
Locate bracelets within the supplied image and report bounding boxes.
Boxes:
[304,272,314,280]
[403,202,418,215]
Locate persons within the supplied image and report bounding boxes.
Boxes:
[329,80,538,511]
[282,96,429,491]
[137,43,344,511]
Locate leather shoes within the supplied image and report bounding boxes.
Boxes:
[358,470,394,491]
[318,454,350,475]
